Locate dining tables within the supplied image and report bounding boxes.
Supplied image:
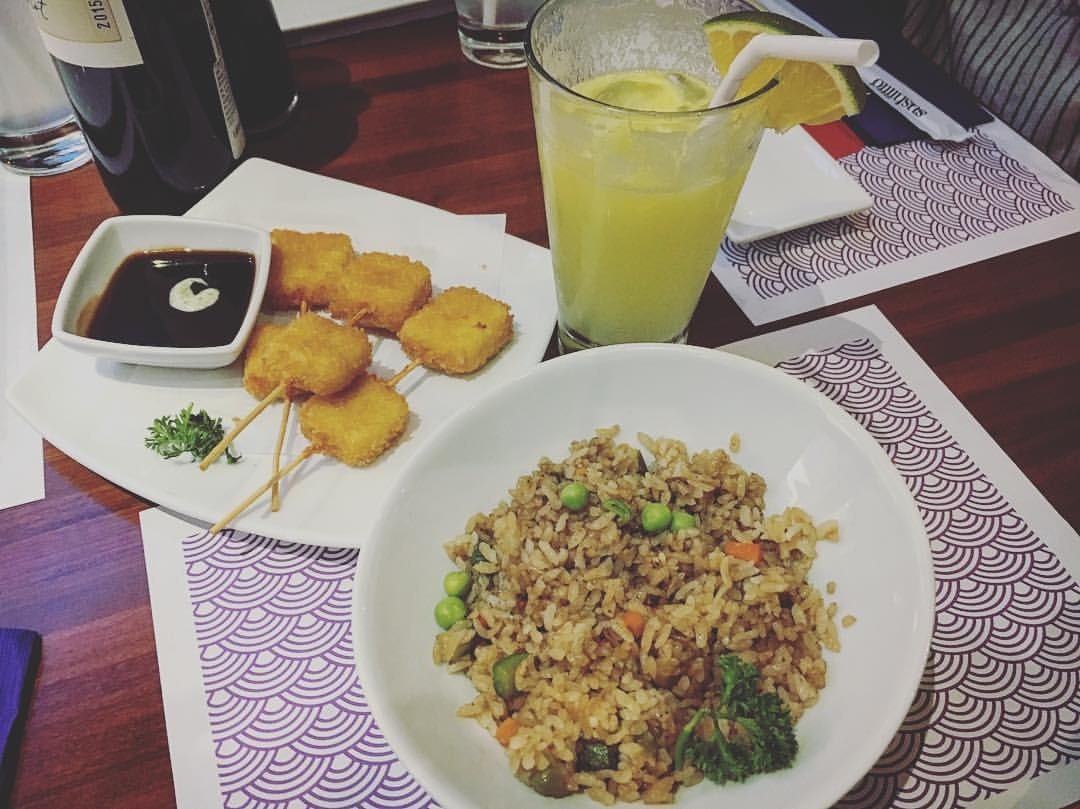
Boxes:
[0,2,1080,802]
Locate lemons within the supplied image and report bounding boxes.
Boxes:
[699,6,869,135]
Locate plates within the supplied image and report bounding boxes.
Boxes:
[725,123,875,244]
[351,342,937,808]
[5,155,556,552]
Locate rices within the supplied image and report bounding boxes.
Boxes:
[428,421,858,804]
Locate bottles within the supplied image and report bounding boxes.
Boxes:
[27,1,310,219]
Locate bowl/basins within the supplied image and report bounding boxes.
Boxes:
[53,215,274,371]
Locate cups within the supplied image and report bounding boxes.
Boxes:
[0,0,94,178]
[523,0,791,360]
[454,0,545,70]
